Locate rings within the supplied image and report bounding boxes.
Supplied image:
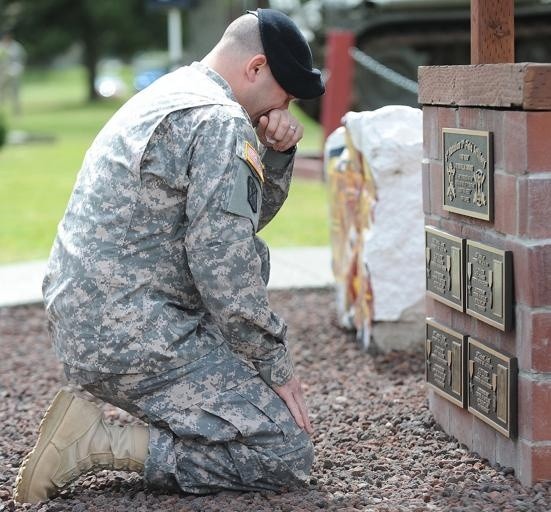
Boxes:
[288,124,298,133]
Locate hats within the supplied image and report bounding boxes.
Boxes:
[256,7,326,101]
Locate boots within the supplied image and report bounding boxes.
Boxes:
[13,387,151,504]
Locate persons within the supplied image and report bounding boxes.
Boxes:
[11,7,327,508]
